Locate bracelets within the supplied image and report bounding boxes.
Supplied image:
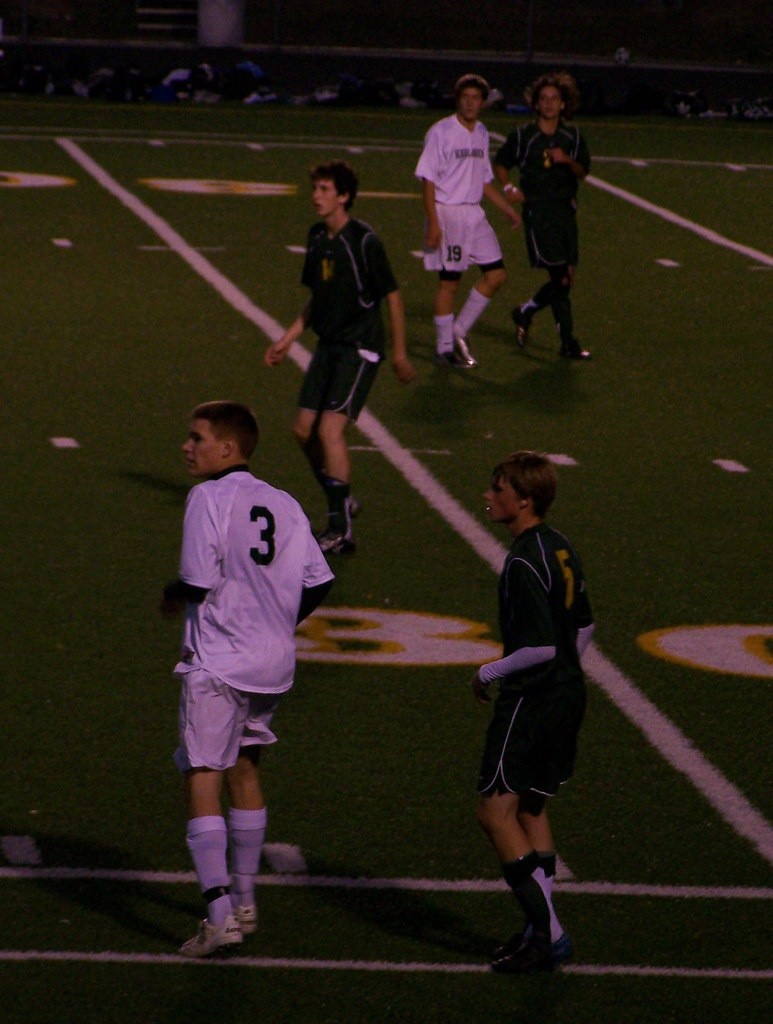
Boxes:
[504,182,512,191]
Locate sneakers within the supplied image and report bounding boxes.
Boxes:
[181,913,242,956]
[233,903,257,934]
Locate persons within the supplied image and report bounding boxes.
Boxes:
[264,161,415,555]
[161,400,335,956]
[492,73,593,360]
[471,451,595,976]
[415,73,523,369]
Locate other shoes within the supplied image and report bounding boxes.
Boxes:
[435,344,479,368]
[511,308,528,347]
[317,531,355,553]
[349,503,362,517]
[493,934,571,972]
[561,344,592,358]
